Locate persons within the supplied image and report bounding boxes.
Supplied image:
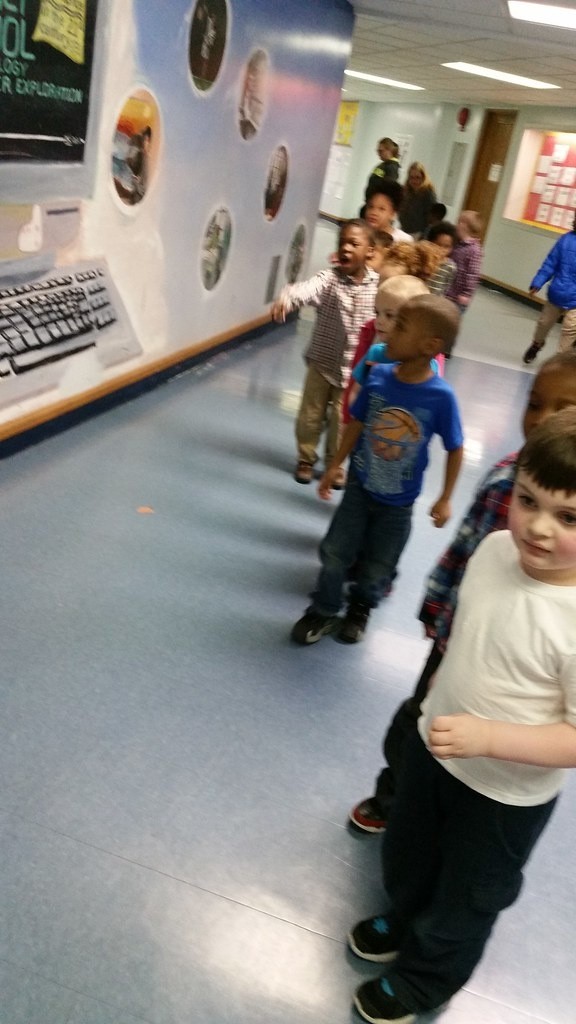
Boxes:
[398,162,436,241]
[271,220,379,488]
[523,207,575,364]
[347,344,576,834]
[132,126,152,205]
[365,230,394,272]
[197,3,216,89]
[290,294,462,645]
[343,239,446,425]
[419,203,446,240]
[349,275,438,425]
[441,210,485,361]
[425,222,459,297]
[364,179,414,242]
[346,405,576,1024]
[363,137,399,217]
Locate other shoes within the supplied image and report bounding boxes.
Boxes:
[353,978,417,1024]
[296,461,312,483]
[524,340,545,364]
[338,603,370,644]
[331,469,346,490]
[348,912,398,963]
[292,605,337,644]
[349,797,386,833]
